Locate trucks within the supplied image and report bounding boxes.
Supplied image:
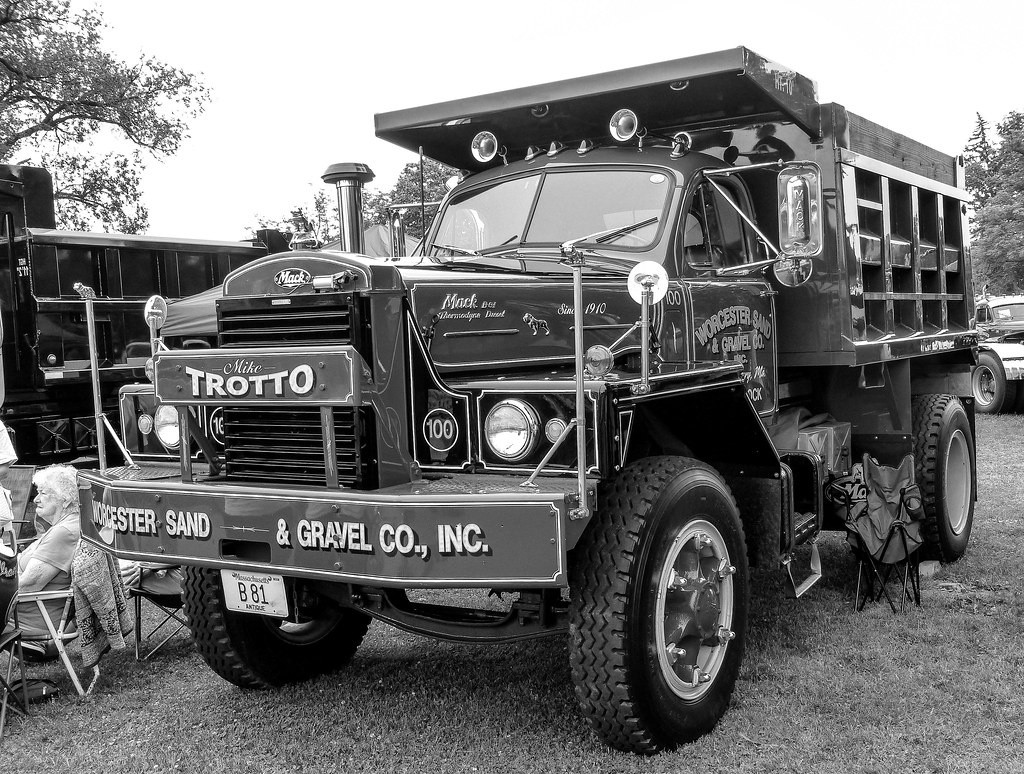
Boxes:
[76,45,980,753]
[972,285,1024,417]
[0,212,289,467]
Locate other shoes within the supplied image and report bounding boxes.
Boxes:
[12,643,60,665]
[7,639,47,659]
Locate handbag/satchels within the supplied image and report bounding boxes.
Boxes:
[9,680,60,703]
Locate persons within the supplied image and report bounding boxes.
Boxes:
[7,463,81,644]
[0,419,18,531]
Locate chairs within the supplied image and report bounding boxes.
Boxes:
[832,450,925,615]
[1,529,189,734]
